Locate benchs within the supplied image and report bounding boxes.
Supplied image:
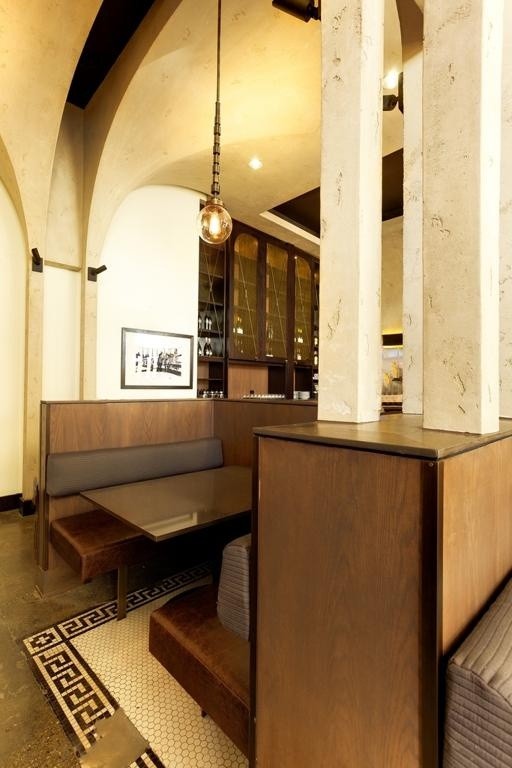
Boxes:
[37,399,216,603]
[145,535,251,767]
[442,573,512,768]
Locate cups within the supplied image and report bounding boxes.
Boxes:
[295,390,311,400]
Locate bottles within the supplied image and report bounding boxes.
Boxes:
[313,335,318,365]
[199,390,224,398]
[232,317,244,334]
[197,336,214,356]
[295,328,303,343]
[198,312,213,330]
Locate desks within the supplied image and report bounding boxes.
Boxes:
[79,463,253,542]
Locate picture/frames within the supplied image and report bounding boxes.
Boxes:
[383,332,404,407]
[121,327,194,389]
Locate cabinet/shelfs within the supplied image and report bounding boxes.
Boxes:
[228,360,318,401]
[230,220,314,368]
[198,235,228,399]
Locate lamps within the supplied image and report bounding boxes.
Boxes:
[197,0,234,244]
[383,67,403,113]
[272,0,321,24]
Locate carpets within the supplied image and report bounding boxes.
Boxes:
[17,557,253,768]
